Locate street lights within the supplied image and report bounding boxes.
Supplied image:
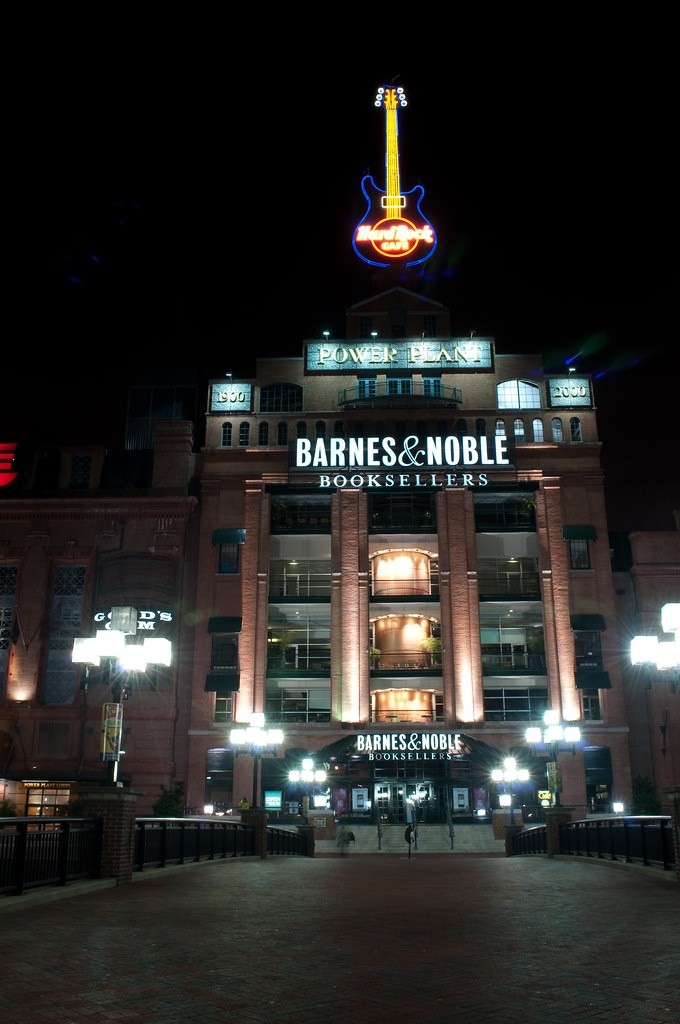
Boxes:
[229,712,284,810]
[525,709,582,809]
[72,605,172,789]
[288,758,327,829]
[491,757,531,825]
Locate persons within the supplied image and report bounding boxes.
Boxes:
[336,826,348,855]
[239,796,250,810]
